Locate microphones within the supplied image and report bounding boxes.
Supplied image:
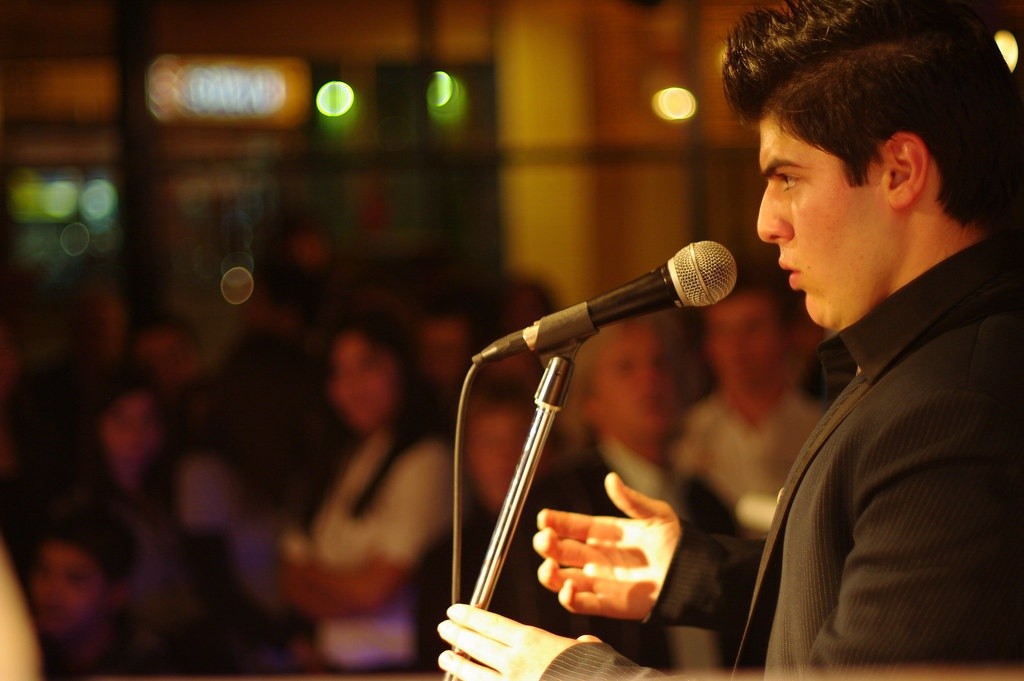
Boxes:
[471,241,738,370]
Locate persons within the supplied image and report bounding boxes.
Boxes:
[437,0,1024,681]
[0,218,827,680]
